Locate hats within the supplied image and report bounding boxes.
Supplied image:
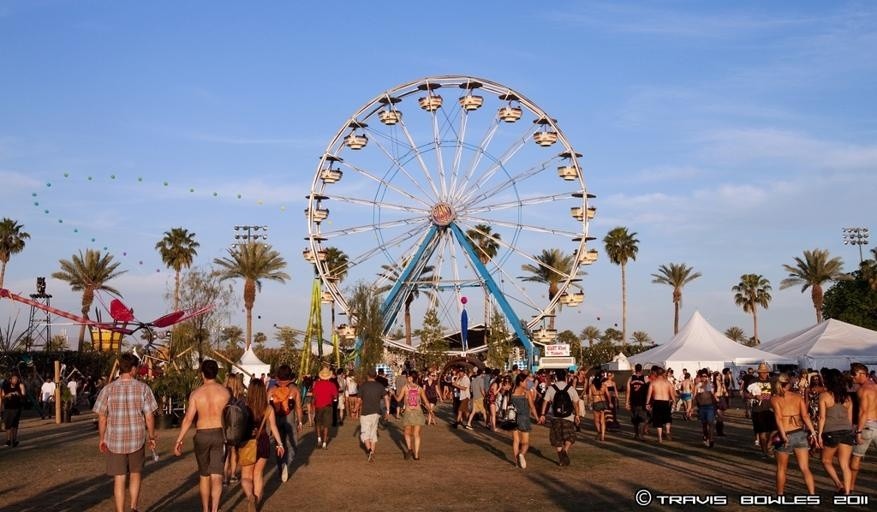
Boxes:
[754,363,771,372]
[318,367,333,380]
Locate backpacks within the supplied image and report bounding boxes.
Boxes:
[218,387,254,446]
[550,383,574,417]
[271,382,290,416]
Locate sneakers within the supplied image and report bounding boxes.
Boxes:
[513,450,525,469]
[317,437,326,449]
[368,448,377,465]
[223,463,289,512]
[558,449,570,467]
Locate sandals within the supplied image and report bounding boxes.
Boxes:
[453,422,502,433]
[405,449,419,460]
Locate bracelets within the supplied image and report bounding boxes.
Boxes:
[175,441,183,444]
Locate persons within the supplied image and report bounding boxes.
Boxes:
[223,359,877,511]
[175,360,231,512]
[91,352,158,512]
[0,371,108,448]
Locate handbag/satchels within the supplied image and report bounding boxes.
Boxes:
[500,403,518,431]
[236,439,257,466]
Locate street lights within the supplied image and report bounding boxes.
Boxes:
[230,224,271,251]
[842,226,870,263]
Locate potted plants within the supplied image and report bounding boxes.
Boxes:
[148,376,182,431]
[60,382,73,423]
